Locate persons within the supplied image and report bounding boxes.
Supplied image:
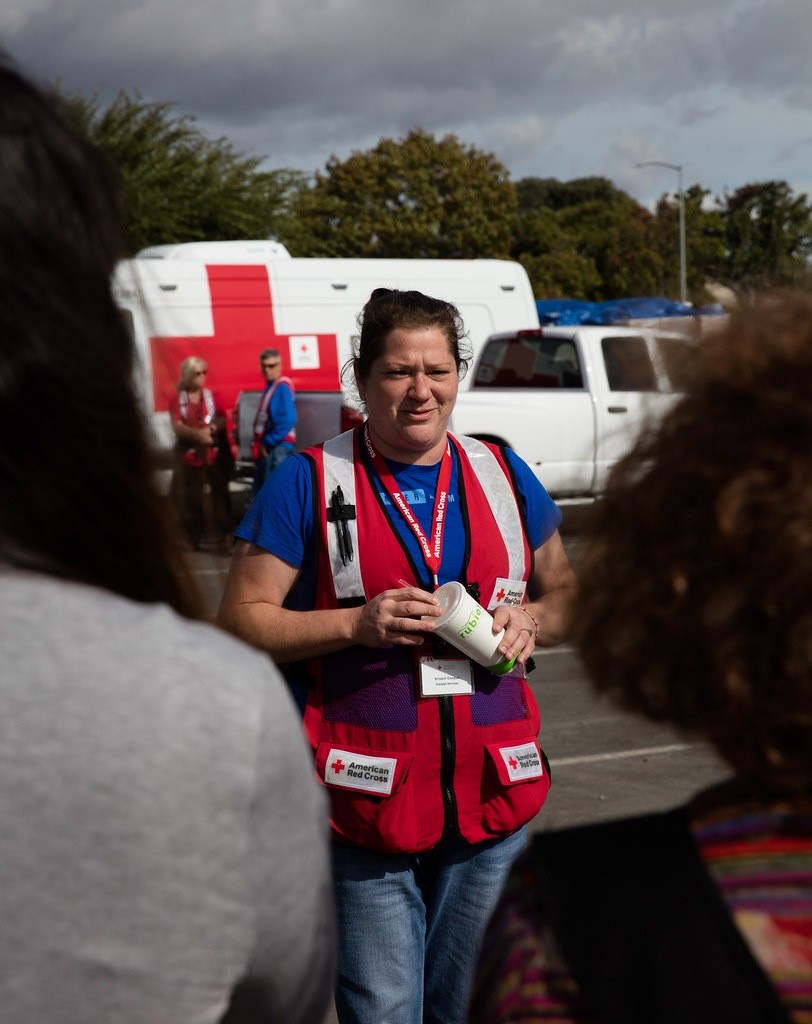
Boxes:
[0,39,812,1024]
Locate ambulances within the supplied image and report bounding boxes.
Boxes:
[93,240,545,466]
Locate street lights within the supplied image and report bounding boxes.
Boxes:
[628,158,687,306]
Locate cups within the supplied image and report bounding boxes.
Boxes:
[422,581,522,677]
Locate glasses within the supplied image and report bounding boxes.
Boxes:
[261,362,280,368]
[196,369,207,376]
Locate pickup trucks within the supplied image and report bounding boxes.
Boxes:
[235,324,714,519]
